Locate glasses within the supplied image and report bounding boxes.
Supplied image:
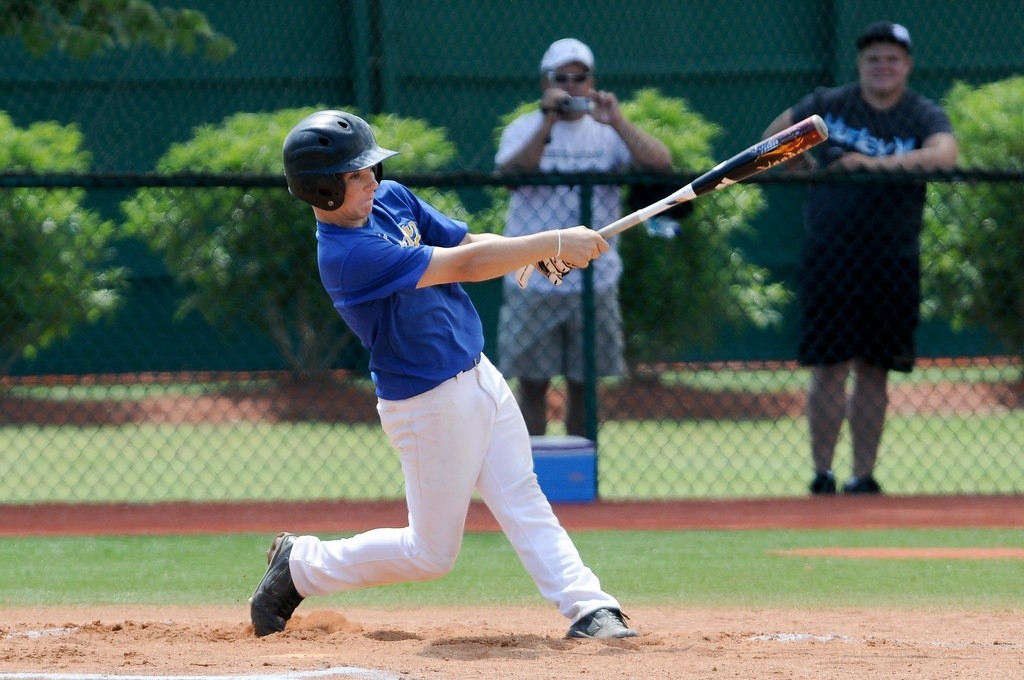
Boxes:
[548,71,590,83]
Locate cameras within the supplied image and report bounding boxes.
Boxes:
[560,95,590,112]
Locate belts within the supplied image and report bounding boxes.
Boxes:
[463,352,481,373]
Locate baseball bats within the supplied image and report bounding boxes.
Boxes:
[595,114,829,241]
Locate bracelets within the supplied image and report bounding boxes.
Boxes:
[555,229,561,258]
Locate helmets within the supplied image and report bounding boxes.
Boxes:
[282,110,400,212]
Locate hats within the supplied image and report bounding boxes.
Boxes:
[855,20,912,53]
[540,37,594,75]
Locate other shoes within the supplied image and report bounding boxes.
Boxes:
[809,472,835,495]
[843,475,881,495]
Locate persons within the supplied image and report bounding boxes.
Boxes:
[760,19,959,496]
[496,36,674,443]
[249,109,662,639]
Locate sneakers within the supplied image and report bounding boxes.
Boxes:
[566,608,637,638]
[248,533,305,637]
